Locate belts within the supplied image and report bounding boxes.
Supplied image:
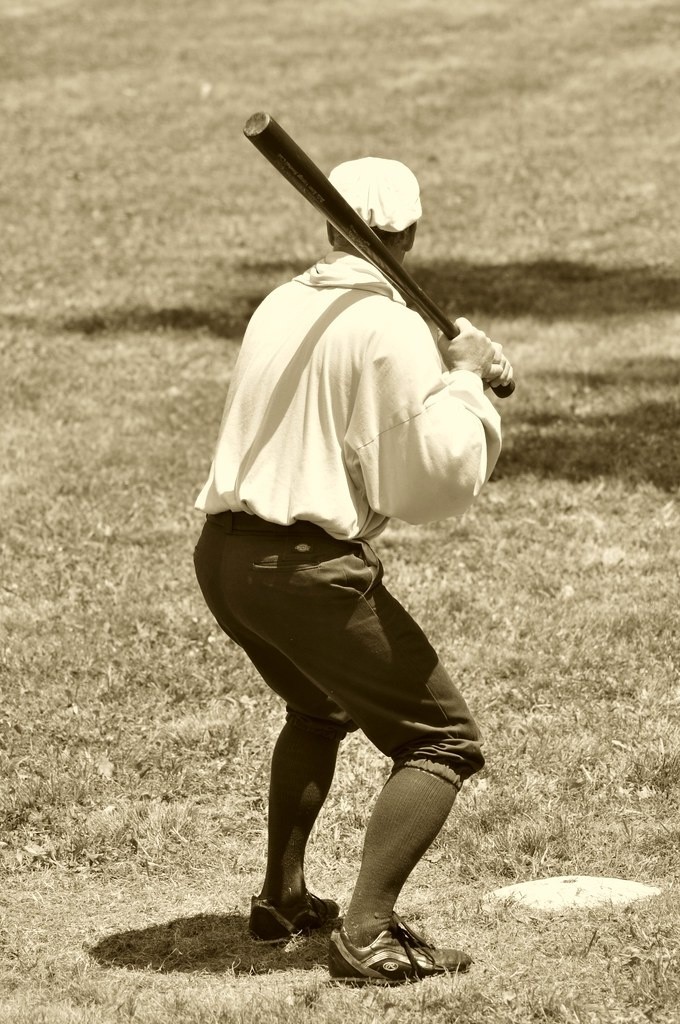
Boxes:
[200,510,319,536]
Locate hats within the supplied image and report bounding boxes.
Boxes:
[325,156,429,234]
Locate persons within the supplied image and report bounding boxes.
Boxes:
[192,153,514,989]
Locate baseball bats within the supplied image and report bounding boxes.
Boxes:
[243,111,516,399]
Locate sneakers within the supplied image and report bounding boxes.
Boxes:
[326,912,473,987]
[249,892,340,944]
